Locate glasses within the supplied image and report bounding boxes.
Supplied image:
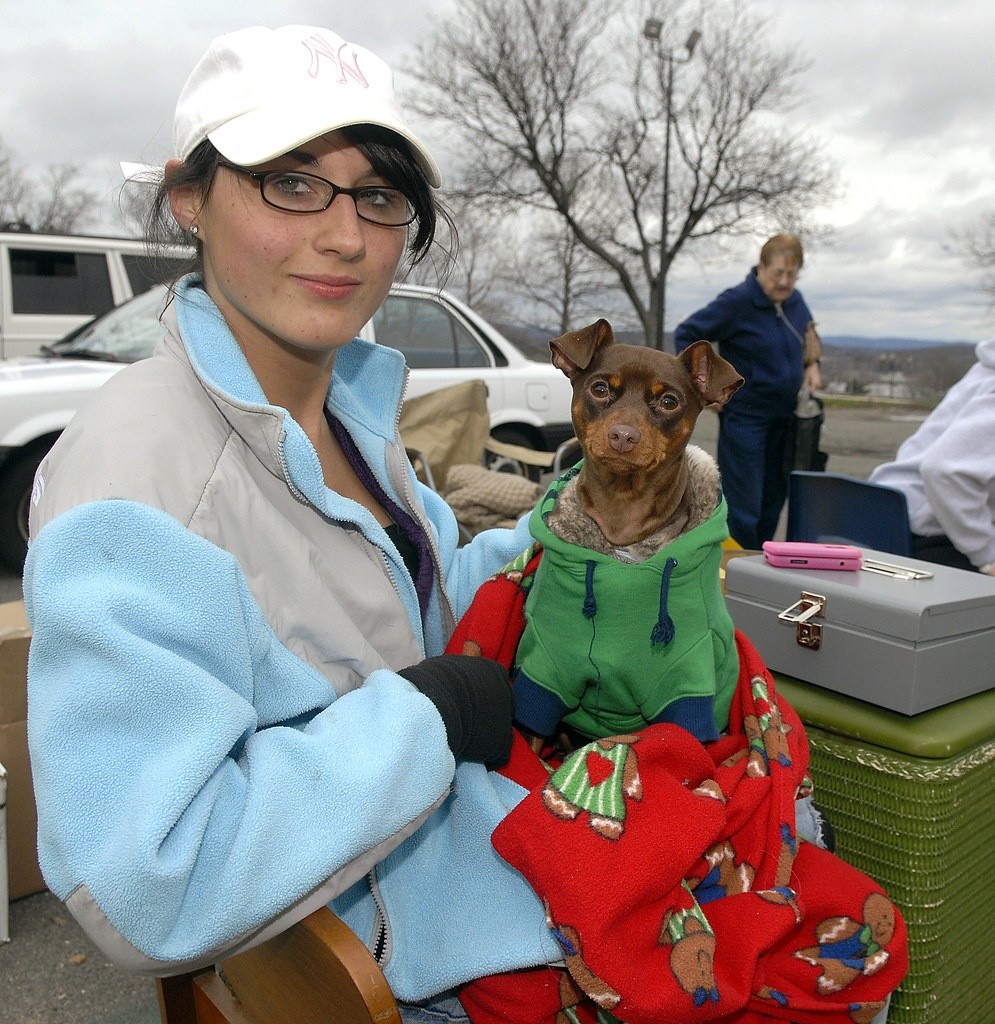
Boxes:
[216,162,419,230]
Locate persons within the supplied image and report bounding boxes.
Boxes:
[20,25,609,1021]
[674,234,824,551]
[864,337,995,577]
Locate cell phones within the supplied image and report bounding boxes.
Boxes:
[762,541,864,571]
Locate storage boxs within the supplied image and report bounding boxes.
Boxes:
[723,545,995,718]
[0,574,47,901]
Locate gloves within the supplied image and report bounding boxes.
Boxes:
[396,654,513,772]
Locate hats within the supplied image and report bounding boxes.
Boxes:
[168,22,445,196]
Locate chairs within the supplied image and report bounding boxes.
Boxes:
[155,904,403,1024]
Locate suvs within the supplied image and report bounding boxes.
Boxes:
[1,231,586,583]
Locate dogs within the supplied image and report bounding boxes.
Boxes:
[512,318,745,761]
